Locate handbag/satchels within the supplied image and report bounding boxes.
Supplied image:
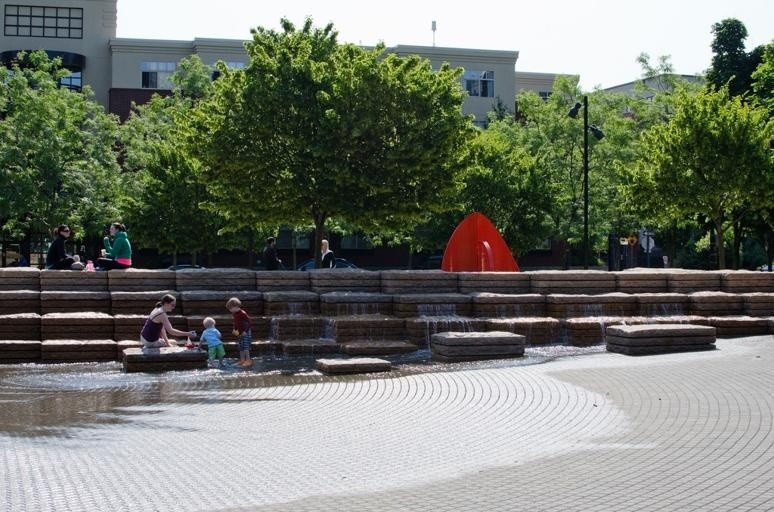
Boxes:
[71,261,85,271]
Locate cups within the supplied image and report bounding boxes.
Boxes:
[73,255,80,261]
[101,249,106,256]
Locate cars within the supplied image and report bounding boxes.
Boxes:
[757,262,774,272]
[168,264,208,271]
[288,256,360,270]
[1,239,21,251]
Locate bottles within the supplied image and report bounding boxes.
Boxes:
[86,260,92,271]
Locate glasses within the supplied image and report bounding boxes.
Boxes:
[61,229,70,233]
[168,301,176,306]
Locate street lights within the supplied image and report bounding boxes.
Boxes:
[568,94,607,267]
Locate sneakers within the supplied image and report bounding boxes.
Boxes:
[206,359,254,367]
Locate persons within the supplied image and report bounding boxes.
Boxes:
[97,222,132,270]
[139,294,198,348]
[262,237,286,270]
[225,297,252,367]
[321,239,336,268]
[197,317,226,369]
[46,224,77,270]
